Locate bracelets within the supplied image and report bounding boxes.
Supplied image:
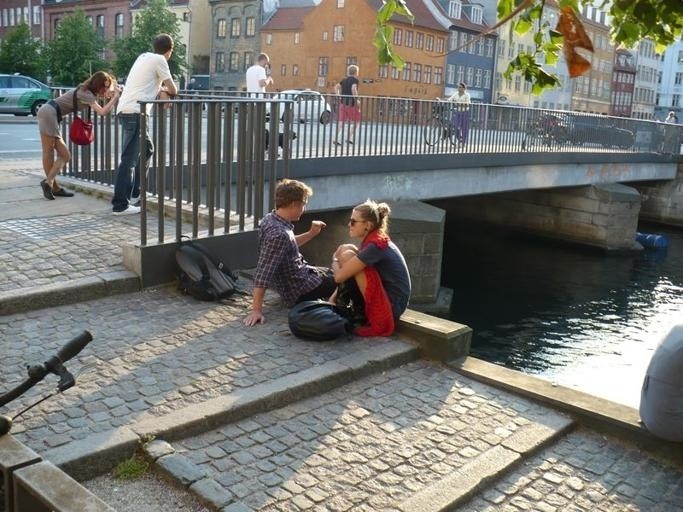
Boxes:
[330,258,339,264]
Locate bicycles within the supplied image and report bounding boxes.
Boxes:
[423,97,473,150]
[1,320,97,512]
[520,116,564,152]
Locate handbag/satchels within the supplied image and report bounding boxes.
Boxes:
[69,117,95,146]
[284,298,356,342]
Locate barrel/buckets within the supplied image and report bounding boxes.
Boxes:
[635,231,669,249]
[641,248,668,264]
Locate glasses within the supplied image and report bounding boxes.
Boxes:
[347,217,370,227]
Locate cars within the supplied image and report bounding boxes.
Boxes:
[533,111,638,152]
[0,71,55,118]
[262,88,335,127]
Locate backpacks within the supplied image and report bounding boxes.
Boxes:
[171,234,240,303]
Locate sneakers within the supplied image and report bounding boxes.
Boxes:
[40,179,74,200]
[329,138,356,148]
[110,187,145,216]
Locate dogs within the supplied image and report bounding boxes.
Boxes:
[265,129,297,157]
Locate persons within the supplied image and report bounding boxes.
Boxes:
[333,65,361,145]
[241,178,337,327]
[36,71,124,200]
[111,32,178,215]
[436,82,470,146]
[245,52,273,104]
[327,200,412,337]
[665,110,676,153]
